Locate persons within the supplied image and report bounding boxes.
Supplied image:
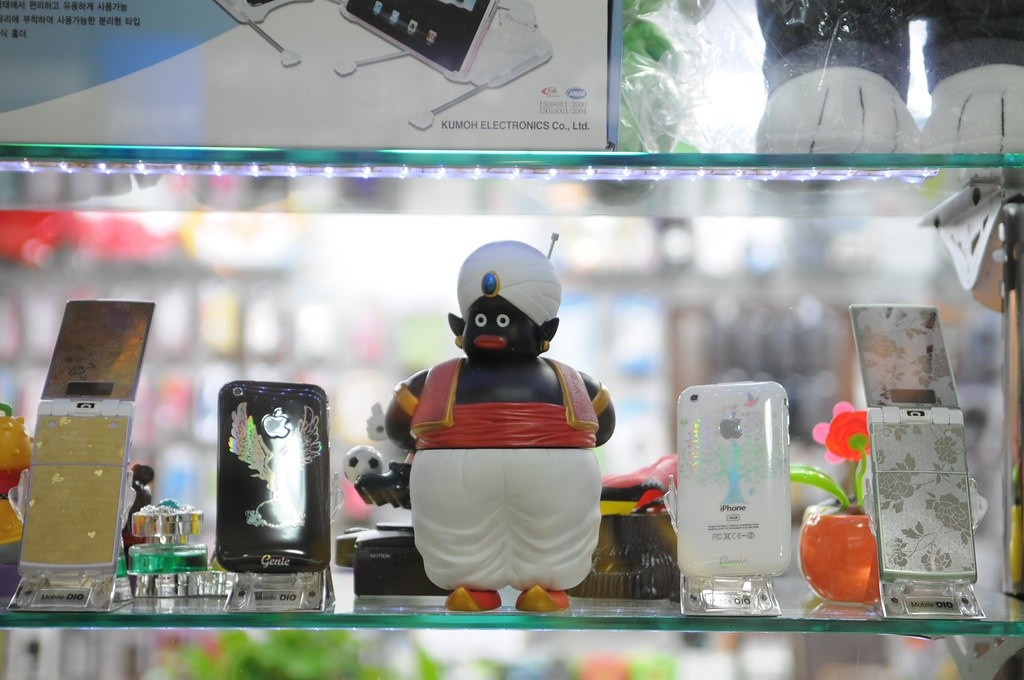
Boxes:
[380,239,616,612]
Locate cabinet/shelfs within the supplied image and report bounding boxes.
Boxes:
[0,142,1023,634]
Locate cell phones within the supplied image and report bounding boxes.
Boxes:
[677,381,793,576]
[848,303,979,586]
[215,379,331,573]
[17,298,155,581]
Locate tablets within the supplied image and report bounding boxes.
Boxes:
[339,0,497,84]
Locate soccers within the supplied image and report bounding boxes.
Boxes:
[342,445,384,485]
